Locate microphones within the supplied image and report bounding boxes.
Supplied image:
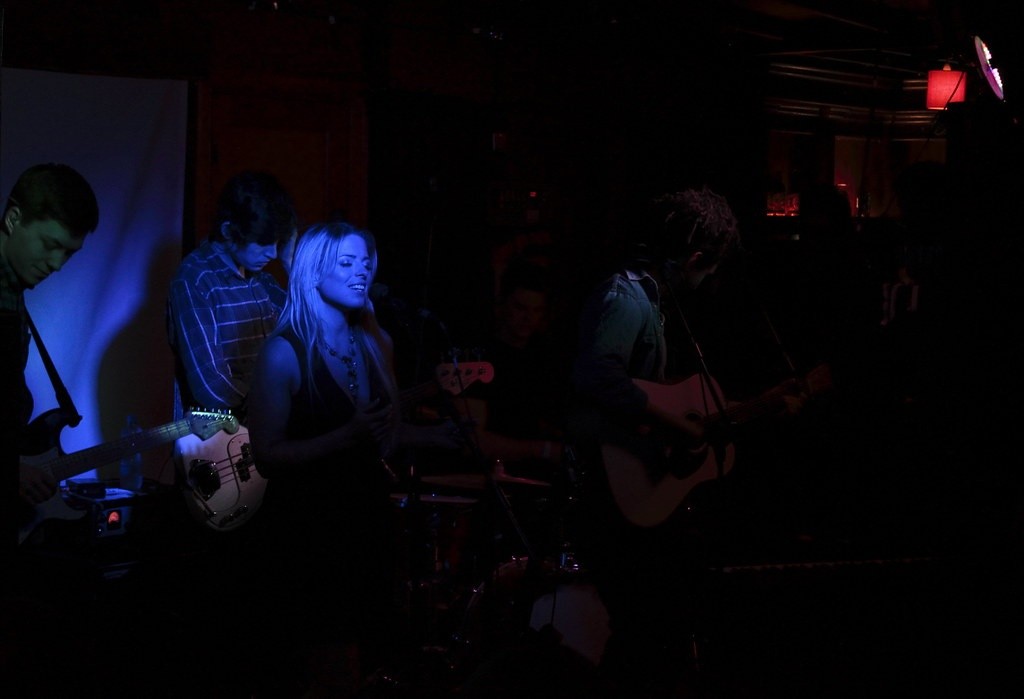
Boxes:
[367,282,435,320]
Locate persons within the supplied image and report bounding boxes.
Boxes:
[162,170,298,508]
[245,221,479,699]
[451,268,570,470]
[1,163,99,545]
[575,185,808,459]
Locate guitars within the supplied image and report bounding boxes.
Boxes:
[597,360,835,529]
[172,346,497,533]
[18,406,241,545]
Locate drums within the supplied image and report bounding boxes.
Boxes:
[389,493,478,575]
[453,555,702,694]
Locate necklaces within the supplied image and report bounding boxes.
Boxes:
[316,323,369,403]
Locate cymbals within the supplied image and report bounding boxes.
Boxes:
[489,469,551,489]
[421,473,488,490]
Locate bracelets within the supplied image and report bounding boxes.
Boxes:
[543,439,551,456]
